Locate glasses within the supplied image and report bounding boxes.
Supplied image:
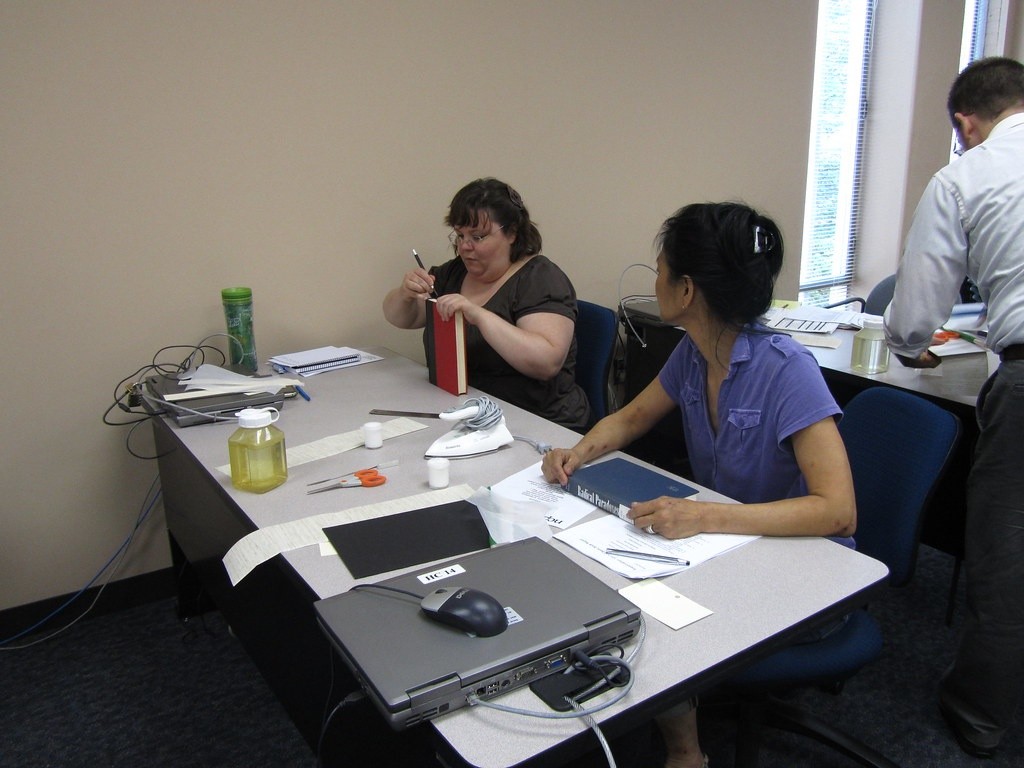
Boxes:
[954,124,964,157]
[449,224,505,247]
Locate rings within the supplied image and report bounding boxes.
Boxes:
[645,525,657,536]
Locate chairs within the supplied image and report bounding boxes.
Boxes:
[700,387,966,768]
[573,301,620,452]
[864,274,962,326]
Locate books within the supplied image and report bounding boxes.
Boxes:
[558,454,702,528]
[424,297,468,397]
[268,345,362,375]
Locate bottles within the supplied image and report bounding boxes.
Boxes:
[221,288,258,376]
[227,406,288,494]
[850,319,890,374]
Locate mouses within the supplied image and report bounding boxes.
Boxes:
[421,587,508,638]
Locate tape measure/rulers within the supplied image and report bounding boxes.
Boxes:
[370,408,440,420]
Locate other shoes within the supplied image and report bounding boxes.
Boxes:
[941,687,997,759]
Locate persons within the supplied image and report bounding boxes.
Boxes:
[380,175,592,440]
[879,54,1024,757]
[538,202,860,768]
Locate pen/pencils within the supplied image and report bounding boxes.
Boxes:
[958,330,988,350]
[412,245,439,296]
[294,383,311,402]
[607,546,691,563]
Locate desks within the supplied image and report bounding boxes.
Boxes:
[132,344,890,768]
[618,290,1001,628]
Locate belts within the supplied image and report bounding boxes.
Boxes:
[999,344,1024,361]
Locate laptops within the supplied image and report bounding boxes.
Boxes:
[145,363,285,426]
[618,300,682,328]
[314,535,643,731]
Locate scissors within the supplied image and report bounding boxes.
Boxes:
[305,469,387,495]
[932,330,961,342]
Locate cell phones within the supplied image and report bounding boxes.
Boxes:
[284,385,298,398]
